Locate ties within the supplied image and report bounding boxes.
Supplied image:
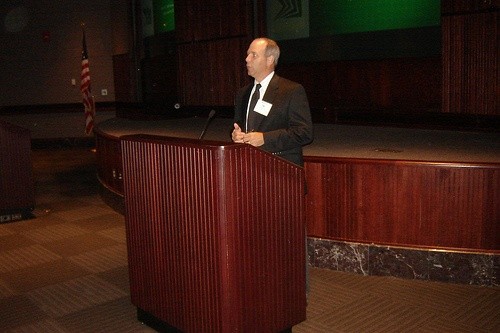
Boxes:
[247,83,262,134]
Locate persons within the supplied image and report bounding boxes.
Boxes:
[230,38,312,194]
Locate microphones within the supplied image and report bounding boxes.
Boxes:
[198,110,216,140]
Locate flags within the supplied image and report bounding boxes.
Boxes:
[79,35,95,136]
